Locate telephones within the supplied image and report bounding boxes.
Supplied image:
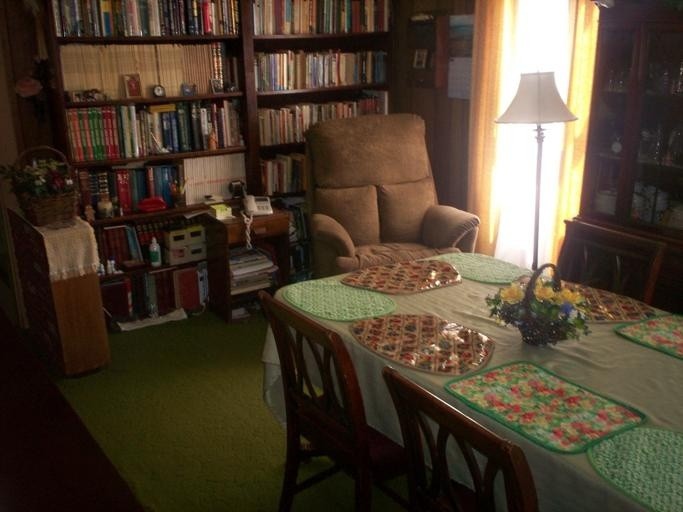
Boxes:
[243,195,273,216]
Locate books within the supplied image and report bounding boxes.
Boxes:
[252,0,388,36]
[71,152,247,217]
[257,89,388,146]
[230,242,284,294]
[253,48,386,93]
[259,152,307,196]
[277,198,312,275]
[99,258,218,322]
[231,290,259,319]
[64,99,240,163]
[51,1,240,37]
[93,217,200,270]
[50,40,238,104]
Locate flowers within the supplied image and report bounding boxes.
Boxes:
[0,155,75,200]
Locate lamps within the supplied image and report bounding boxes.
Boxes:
[494,71,579,269]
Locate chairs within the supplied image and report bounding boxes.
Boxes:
[556,219,668,305]
[379,361,538,512]
[257,290,400,512]
[306,114,481,278]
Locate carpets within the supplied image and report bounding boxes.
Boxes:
[20,307,431,512]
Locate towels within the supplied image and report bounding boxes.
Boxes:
[289,275,495,379]
[559,279,682,360]
[339,252,522,293]
[444,359,683,512]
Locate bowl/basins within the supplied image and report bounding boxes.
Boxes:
[594,189,616,215]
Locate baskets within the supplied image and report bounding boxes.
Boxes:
[503,262,577,348]
[10,146,79,228]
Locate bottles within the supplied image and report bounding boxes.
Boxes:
[651,59,683,169]
[148,236,162,269]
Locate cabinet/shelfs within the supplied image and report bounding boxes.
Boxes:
[48,0,393,328]
[206,210,290,325]
[579,0,683,315]
